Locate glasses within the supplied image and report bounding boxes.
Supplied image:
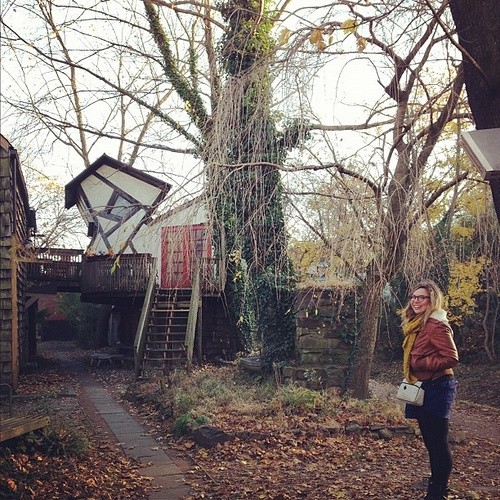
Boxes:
[410,295,431,302]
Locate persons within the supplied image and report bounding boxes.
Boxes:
[398,280,460,499]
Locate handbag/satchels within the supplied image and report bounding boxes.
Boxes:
[396,377,425,406]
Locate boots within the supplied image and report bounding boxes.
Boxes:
[422,477,432,500]
[431,479,447,500]
